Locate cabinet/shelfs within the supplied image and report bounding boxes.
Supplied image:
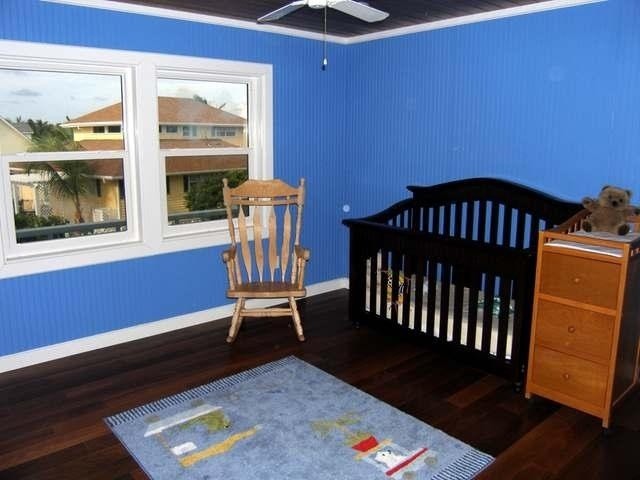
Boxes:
[525,198,640,428]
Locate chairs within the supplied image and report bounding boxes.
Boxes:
[220,178,310,345]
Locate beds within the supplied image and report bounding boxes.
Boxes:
[340,177,589,393]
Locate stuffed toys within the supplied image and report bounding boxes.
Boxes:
[581,185,640,236]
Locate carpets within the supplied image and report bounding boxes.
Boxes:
[102,352,496,478]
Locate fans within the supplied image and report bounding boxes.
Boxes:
[255,1,391,68]
